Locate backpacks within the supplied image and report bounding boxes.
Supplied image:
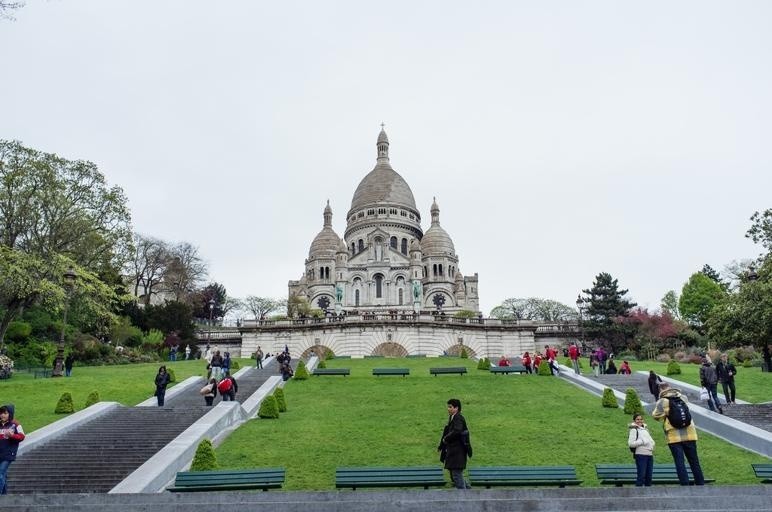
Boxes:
[660,395,692,429]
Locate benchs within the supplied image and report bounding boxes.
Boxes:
[489,365,528,376]
[313,368,351,377]
[166,465,287,495]
[429,366,468,377]
[751,462,771,484]
[593,460,717,491]
[333,464,449,492]
[372,368,411,379]
[466,463,584,489]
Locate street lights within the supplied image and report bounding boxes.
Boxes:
[52,264,77,376]
[206,298,216,357]
[578,305,593,357]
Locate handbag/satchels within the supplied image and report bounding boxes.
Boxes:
[199,380,215,396]
[217,376,233,393]
[700,387,710,403]
[628,427,639,453]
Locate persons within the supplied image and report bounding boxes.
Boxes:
[0,404,25,495]
[64,352,75,377]
[171,341,298,406]
[155,365,170,406]
[439,399,472,489]
[499,341,737,488]
